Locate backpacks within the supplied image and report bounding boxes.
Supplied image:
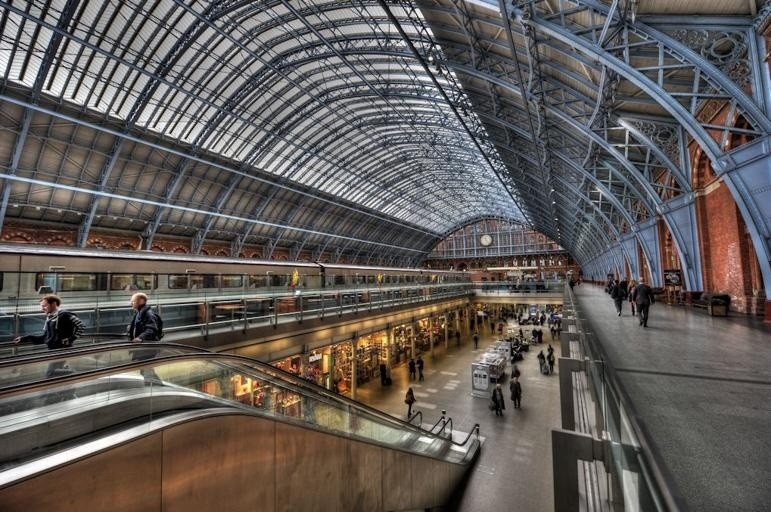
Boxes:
[153,313,164,342]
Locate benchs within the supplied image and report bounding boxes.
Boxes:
[648,286,731,317]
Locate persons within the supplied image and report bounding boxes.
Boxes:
[531,313,562,376]
[511,365,520,379]
[490,321,495,336]
[569,280,575,291]
[473,331,479,349]
[491,383,506,416]
[417,356,424,382]
[13,295,86,406]
[456,331,460,347]
[405,388,417,419]
[409,359,416,381]
[126,292,164,387]
[605,277,655,327]
[519,328,523,337]
[509,377,522,408]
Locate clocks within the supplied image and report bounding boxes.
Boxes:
[480,234,492,247]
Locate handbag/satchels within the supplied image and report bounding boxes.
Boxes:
[628,293,632,302]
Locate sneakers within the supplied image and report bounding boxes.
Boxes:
[639,320,648,327]
[618,312,621,316]
